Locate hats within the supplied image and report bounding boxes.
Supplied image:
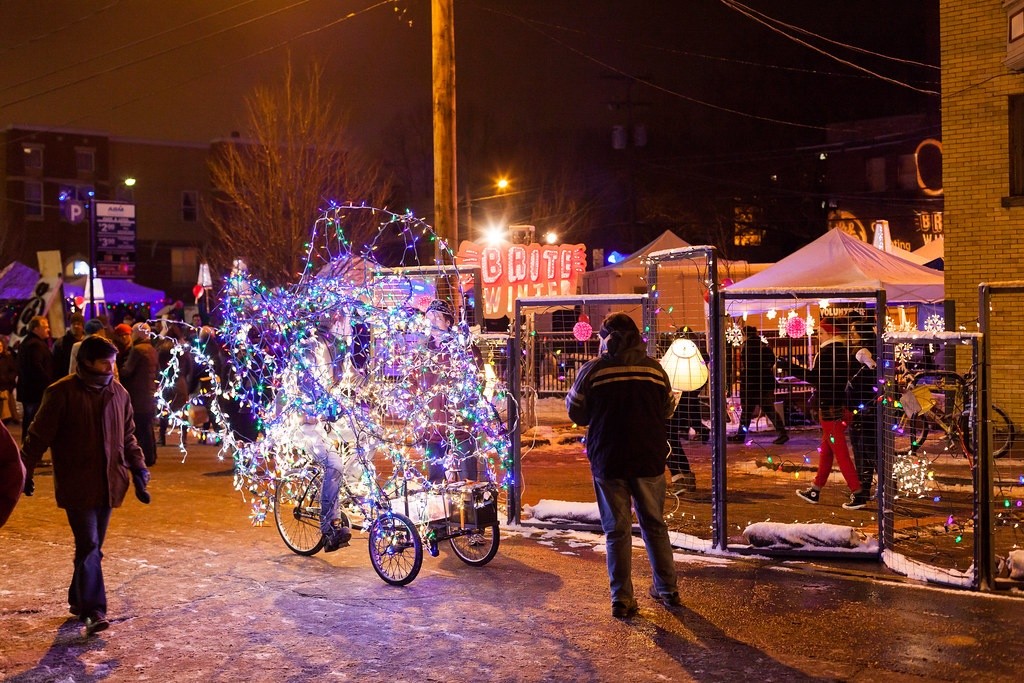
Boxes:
[131,322,151,340]
[819,316,844,336]
[115,324,132,335]
[84,319,107,335]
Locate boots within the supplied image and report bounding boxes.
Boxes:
[684,472,696,491]
[666,475,685,495]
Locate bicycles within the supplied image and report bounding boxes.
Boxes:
[892,362,1015,459]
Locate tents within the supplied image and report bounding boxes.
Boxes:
[1,261,165,321]
[704,227,945,371]
[579,228,776,331]
[314,254,435,311]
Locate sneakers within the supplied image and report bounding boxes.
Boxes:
[842,491,867,509]
[795,487,820,504]
[649,584,681,606]
[611,598,637,617]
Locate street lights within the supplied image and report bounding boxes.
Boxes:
[465,174,510,243]
[87,173,137,319]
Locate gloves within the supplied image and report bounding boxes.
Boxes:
[131,468,150,487]
[776,357,787,369]
[23,478,35,496]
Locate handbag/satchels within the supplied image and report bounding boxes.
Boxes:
[188,403,210,427]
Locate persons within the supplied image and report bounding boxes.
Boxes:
[0,314,278,467]
[410,300,486,539]
[19,335,150,633]
[775,310,884,510]
[565,313,679,617]
[289,312,376,553]
[665,428,695,497]
[0,420,27,529]
[727,326,790,445]
[674,326,710,447]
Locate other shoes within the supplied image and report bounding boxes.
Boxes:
[435,526,447,539]
[86,617,109,635]
[702,428,710,444]
[155,439,166,446]
[69,606,89,622]
[773,435,790,445]
[323,525,351,548]
[678,430,690,440]
[468,533,486,546]
[846,488,871,499]
[37,458,51,467]
[727,434,746,443]
[254,469,265,475]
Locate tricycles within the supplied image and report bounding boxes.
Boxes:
[273,406,502,587]
